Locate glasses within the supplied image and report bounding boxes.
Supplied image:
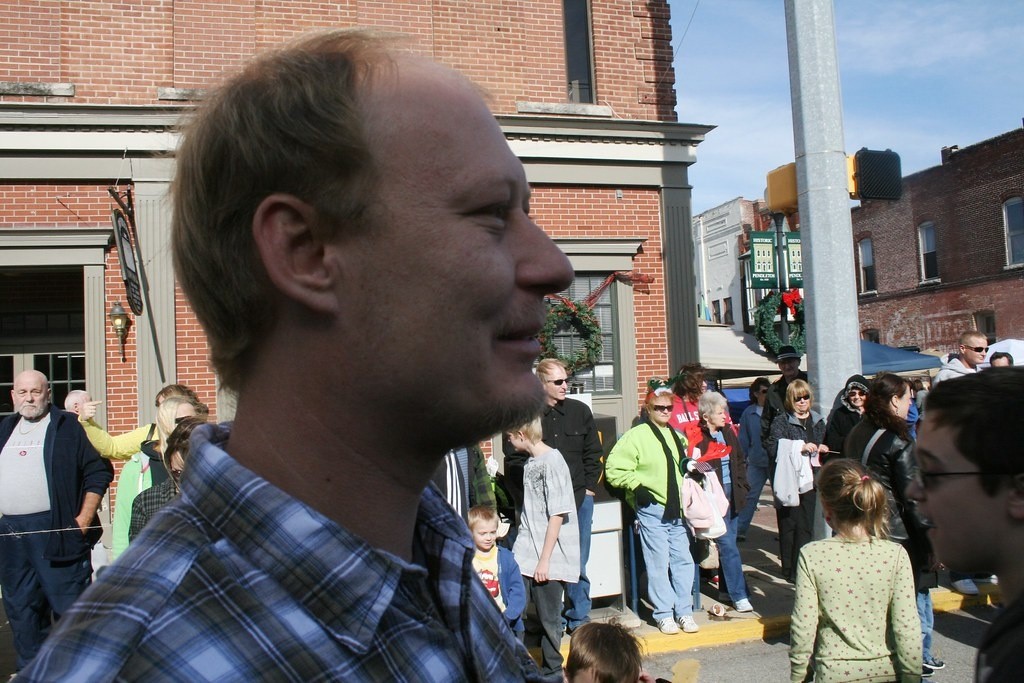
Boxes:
[907,465,986,490]
[171,470,181,479]
[762,389,767,393]
[175,416,190,424]
[546,379,568,386]
[653,404,674,411]
[965,345,989,353]
[794,394,810,402]
[848,391,866,396]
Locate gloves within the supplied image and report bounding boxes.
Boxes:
[634,485,657,509]
[687,469,704,484]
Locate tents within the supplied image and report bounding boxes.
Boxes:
[719,340,948,391]
[976,337,1024,368]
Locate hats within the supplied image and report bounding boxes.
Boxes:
[844,375,871,399]
[775,346,801,363]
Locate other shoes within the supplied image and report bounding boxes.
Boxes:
[922,654,945,669]
[707,575,719,584]
[784,572,795,584]
[736,532,746,541]
[921,665,935,676]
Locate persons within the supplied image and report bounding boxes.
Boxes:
[127,418,206,544]
[430,441,496,525]
[504,360,606,635]
[79,379,200,460]
[65,389,93,413]
[563,621,671,683]
[0,370,115,683]
[18,36,573,683]
[506,417,580,683]
[465,506,524,646]
[604,331,1024,683]
[112,398,196,564]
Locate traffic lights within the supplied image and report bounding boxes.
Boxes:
[846,146,904,202]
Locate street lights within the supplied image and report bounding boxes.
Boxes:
[762,185,791,346]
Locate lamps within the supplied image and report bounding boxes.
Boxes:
[107,301,129,355]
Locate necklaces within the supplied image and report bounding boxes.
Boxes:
[18,418,45,435]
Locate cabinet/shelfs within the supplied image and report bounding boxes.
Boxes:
[585,500,626,611]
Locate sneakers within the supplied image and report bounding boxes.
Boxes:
[974,575,999,585]
[733,598,753,612]
[675,616,699,633]
[951,578,979,595]
[657,616,678,634]
